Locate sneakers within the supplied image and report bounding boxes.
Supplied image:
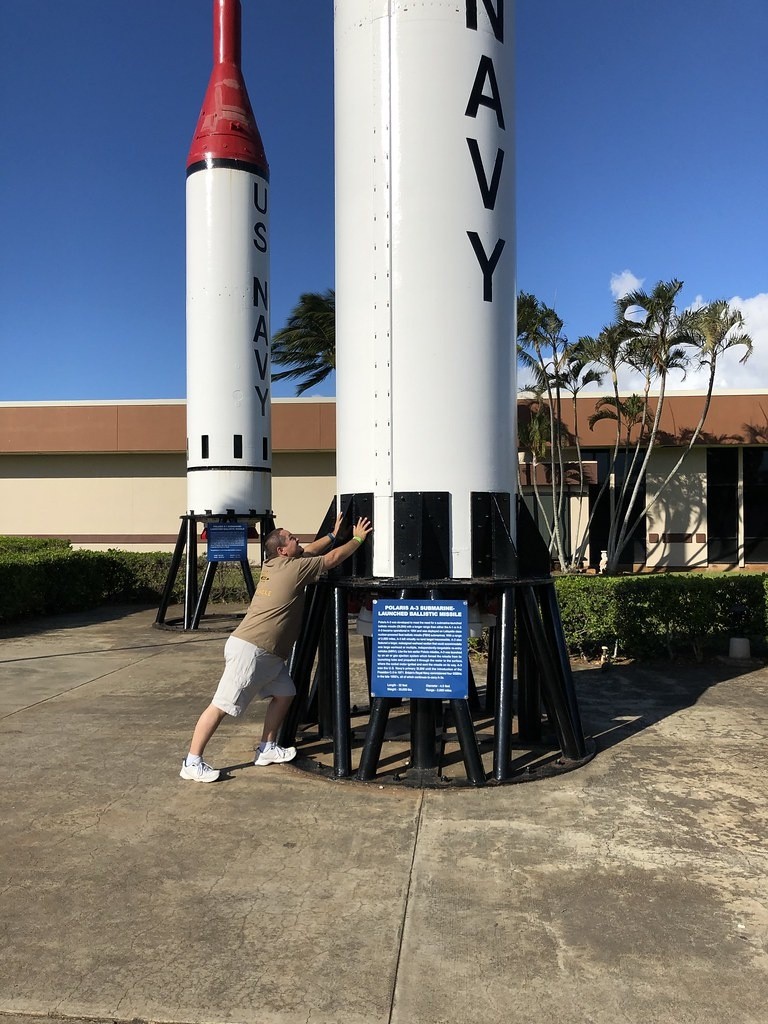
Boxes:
[179,756,221,783]
[253,741,297,766]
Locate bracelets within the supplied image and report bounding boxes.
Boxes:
[328,532,336,540]
[353,536,364,544]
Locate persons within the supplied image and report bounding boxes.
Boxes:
[179,511,373,783]
